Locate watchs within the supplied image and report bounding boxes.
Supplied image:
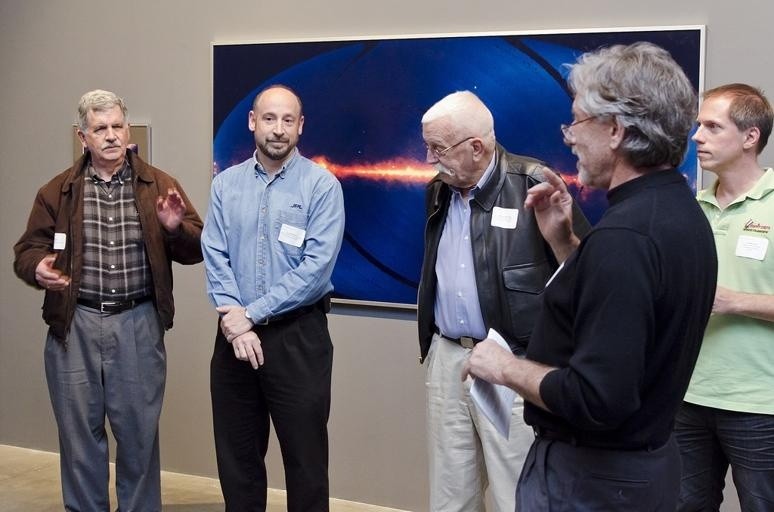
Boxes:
[240,308,255,324]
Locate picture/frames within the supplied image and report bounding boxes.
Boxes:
[72,124,151,165]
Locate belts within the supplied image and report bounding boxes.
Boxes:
[253,302,323,327]
[76,292,151,315]
[431,324,483,349]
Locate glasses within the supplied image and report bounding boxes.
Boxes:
[424,137,473,157]
[561,114,603,145]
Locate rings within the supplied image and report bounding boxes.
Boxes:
[47,285,50,289]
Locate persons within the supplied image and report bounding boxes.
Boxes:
[415,89,592,512]
[200,84,346,511]
[460,41,716,511]
[672,83,774,510]
[12,89,204,512]
